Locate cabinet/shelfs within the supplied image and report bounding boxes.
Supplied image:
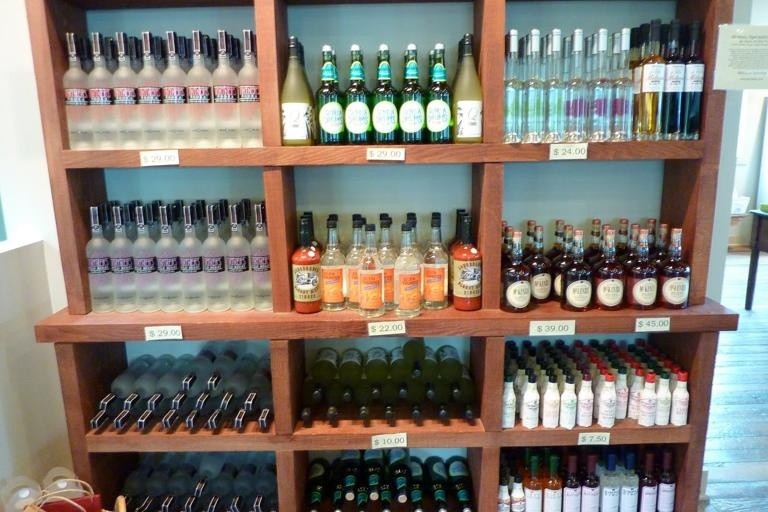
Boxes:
[22,0,739,512]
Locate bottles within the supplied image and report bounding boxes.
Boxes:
[279,22,486,152]
[298,450,479,512]
[501,213,694,310]
[64,21,265,157]
[295,203,485,315]
[300,336,476,432]
[72,198,273,318]
[503,341,695,431]
[505,22,702,144]
[94,452,279,512]
[80,343,275,437]
[499,448,679,510]
